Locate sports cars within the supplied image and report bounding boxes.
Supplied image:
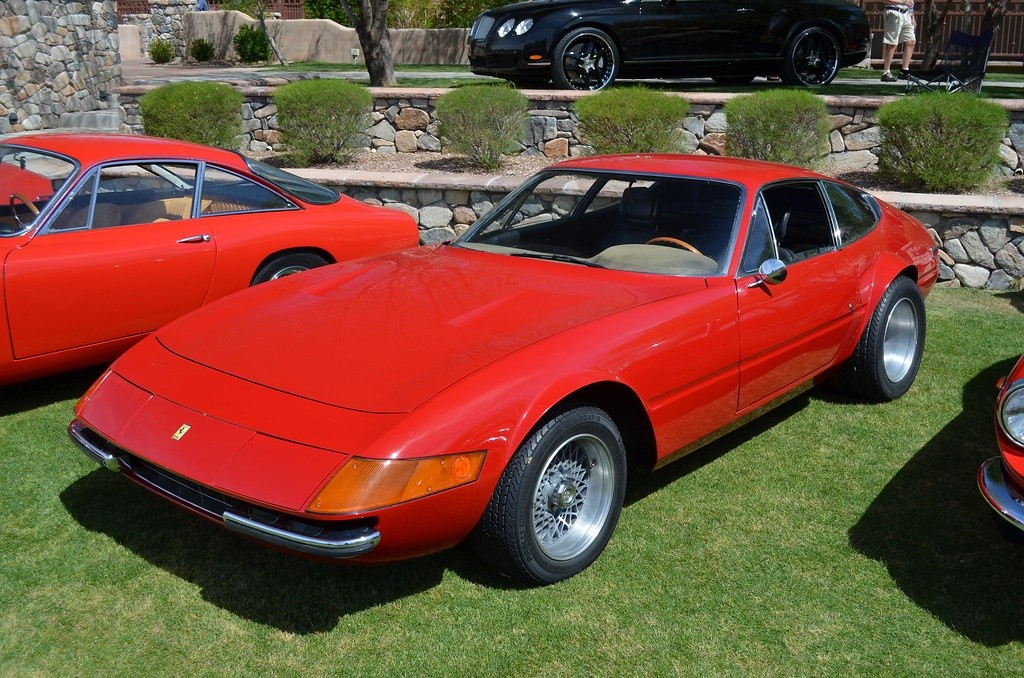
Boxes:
[65,150,943,590]
[0,131,419,394]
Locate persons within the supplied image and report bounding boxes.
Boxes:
[882,0,919,81]
[194,0,208,11]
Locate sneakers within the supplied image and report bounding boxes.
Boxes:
[898,69,920,81]
[880,72,897,82]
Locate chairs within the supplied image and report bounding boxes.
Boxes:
[769,201,795,260]
[899,29,995,96]
[596,187,661,251]
[64,202,122,228]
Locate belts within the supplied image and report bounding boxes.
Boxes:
[886,7,908,14]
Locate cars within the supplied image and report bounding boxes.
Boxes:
[463,1,873,92]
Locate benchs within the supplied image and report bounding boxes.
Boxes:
[128,196,253,224]
